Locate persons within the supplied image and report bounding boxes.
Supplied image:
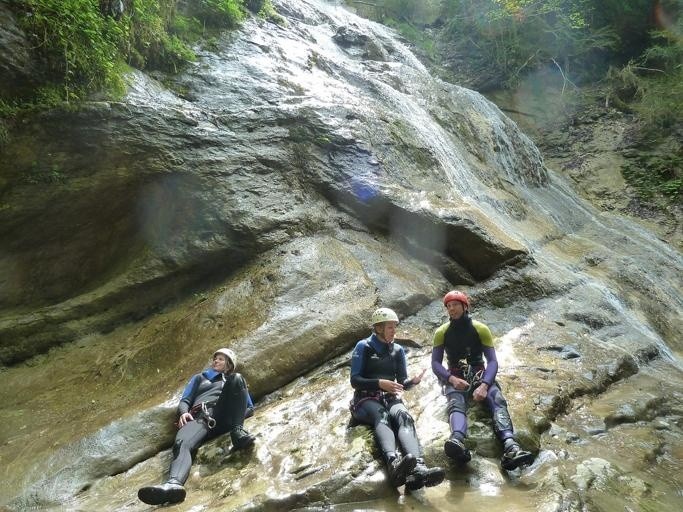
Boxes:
[348,307,446,490]
[138,347,256,505]
[432,289,534,471]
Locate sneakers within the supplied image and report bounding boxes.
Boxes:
[138,482,186,505]
[230,426,255,448]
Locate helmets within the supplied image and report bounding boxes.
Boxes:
[372,308,399,325]
[444,290,469,307]
[214,348,237,370]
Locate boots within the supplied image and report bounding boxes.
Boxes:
[405,458,445,490]
[388,454,417,487]
[444,437,471,464]
[501,441,534,471]
